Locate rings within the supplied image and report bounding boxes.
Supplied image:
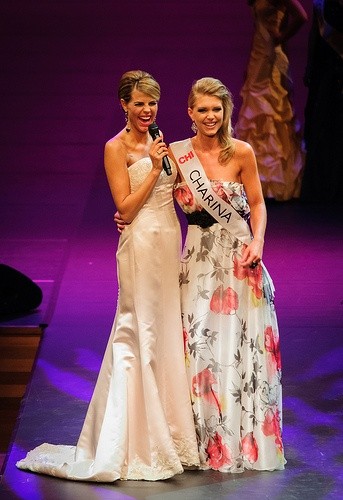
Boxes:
[249,260,258,269]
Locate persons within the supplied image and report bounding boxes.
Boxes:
[113,77,288,473]
[232,1,309,202]
[15,71,203,483]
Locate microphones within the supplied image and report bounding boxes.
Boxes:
[148,124,172,176]
